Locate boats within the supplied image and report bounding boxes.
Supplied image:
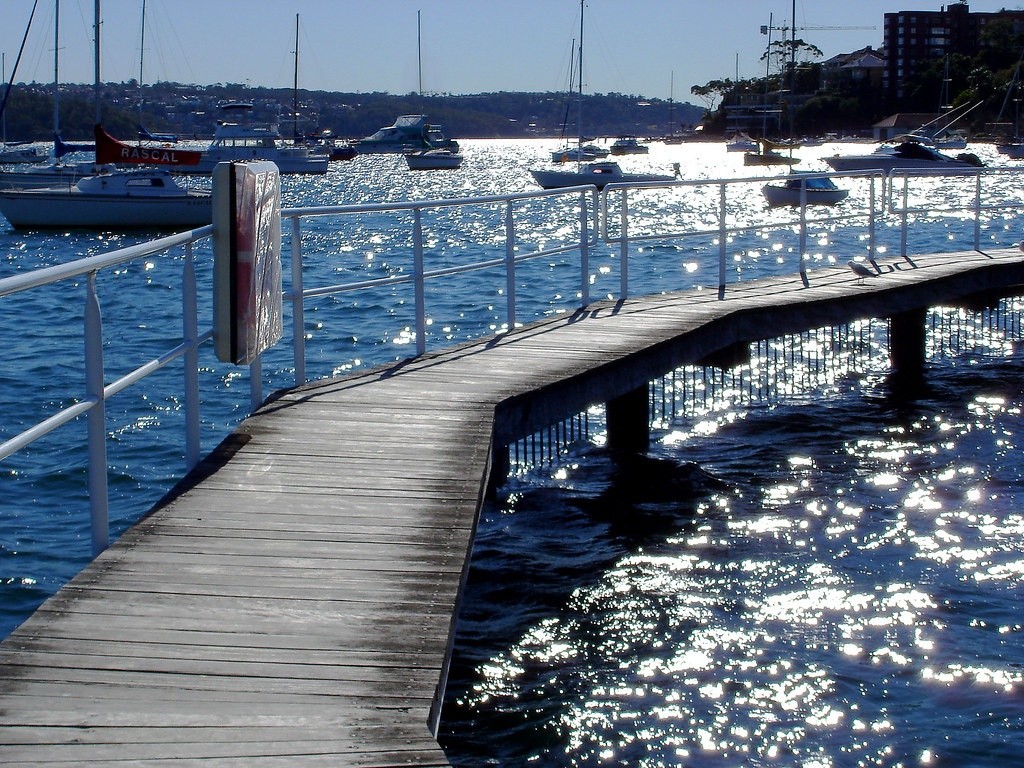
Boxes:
[402,150,462,171]
[761,168,858,205]
[1,164,212,228]
[610,133,650,155]
[816,98,991,176]
[527,160,676,190]
[92,119,330,175]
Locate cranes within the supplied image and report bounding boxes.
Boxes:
[760,18,877,74]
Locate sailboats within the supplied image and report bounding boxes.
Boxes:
[931,53,967,150]
[0,0,115,189]
[290,9,357,160]
[550,35,595,161]
[743,12,824,166]
[989,54,1024,159]
[353,9,459,152]
[572,46,609,158]
[726,51,760,152]
[663,70,683,145]
